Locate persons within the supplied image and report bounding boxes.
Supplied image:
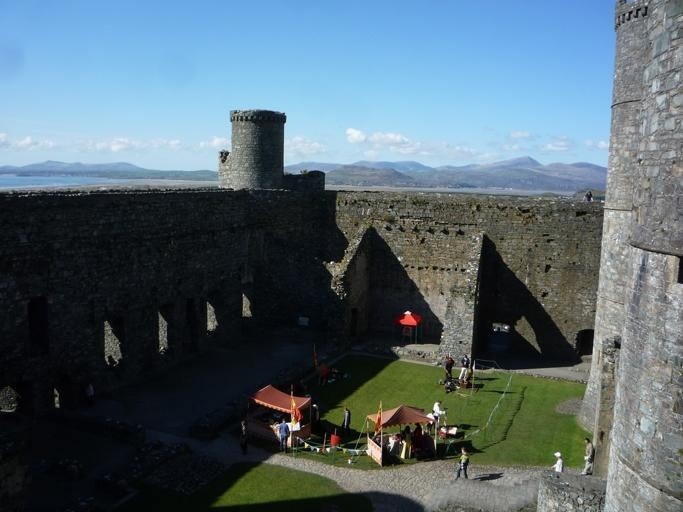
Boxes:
[243,362,350,455]
[582,437,594,475]
[444,354,470,384]
[388,401,446,459]
[455,448,469,480]
[583,190,594,201]
[552,452,564,473]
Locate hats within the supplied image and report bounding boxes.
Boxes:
[554,452,561,458]
